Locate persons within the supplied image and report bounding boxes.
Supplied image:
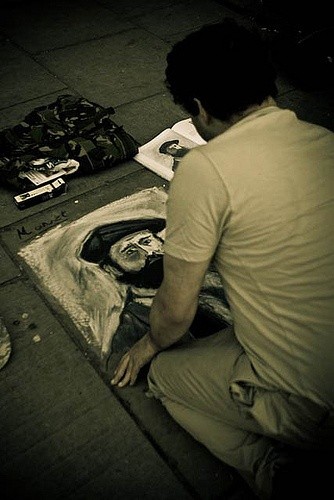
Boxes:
[158,140,194,171]
[109,23,334,500]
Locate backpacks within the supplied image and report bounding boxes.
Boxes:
[0,94,140,190]
